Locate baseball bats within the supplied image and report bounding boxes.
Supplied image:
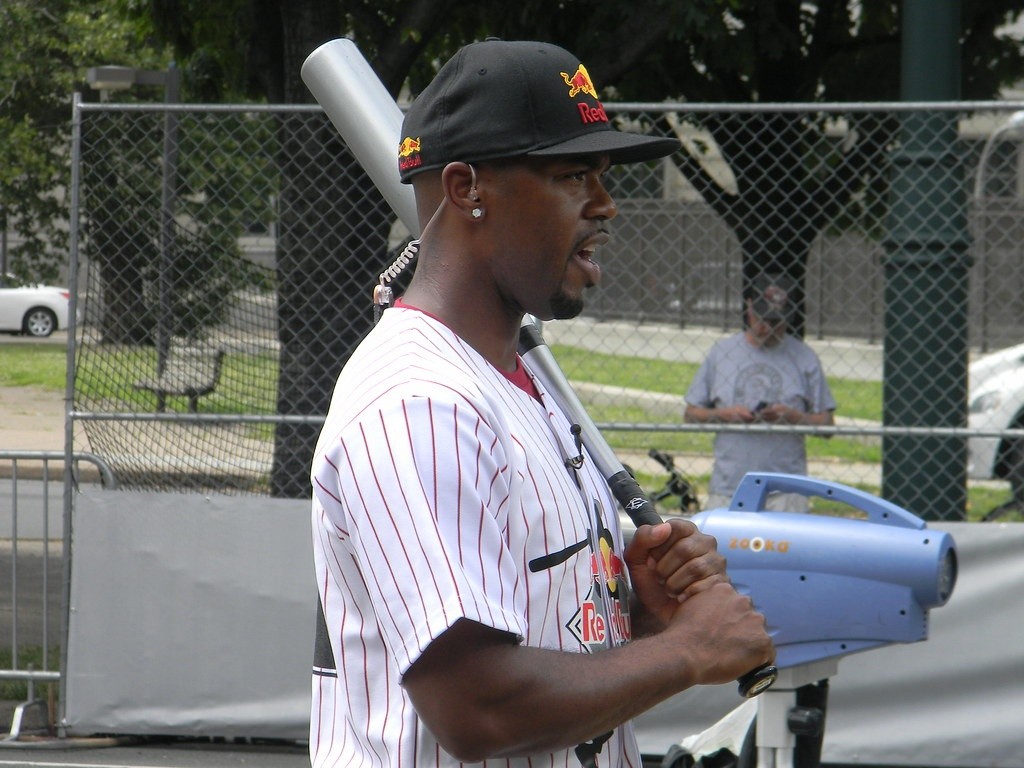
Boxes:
[301,36,780,701]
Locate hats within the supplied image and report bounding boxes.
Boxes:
[398,37,681,184]
[751,274,796,322]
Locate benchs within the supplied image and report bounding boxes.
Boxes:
[134,344,226,414]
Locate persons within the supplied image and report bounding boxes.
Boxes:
[307,41,776,768]
[685,274,837,513]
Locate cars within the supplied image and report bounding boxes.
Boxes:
[968,344,1023,509]
[0,273,81,337]
